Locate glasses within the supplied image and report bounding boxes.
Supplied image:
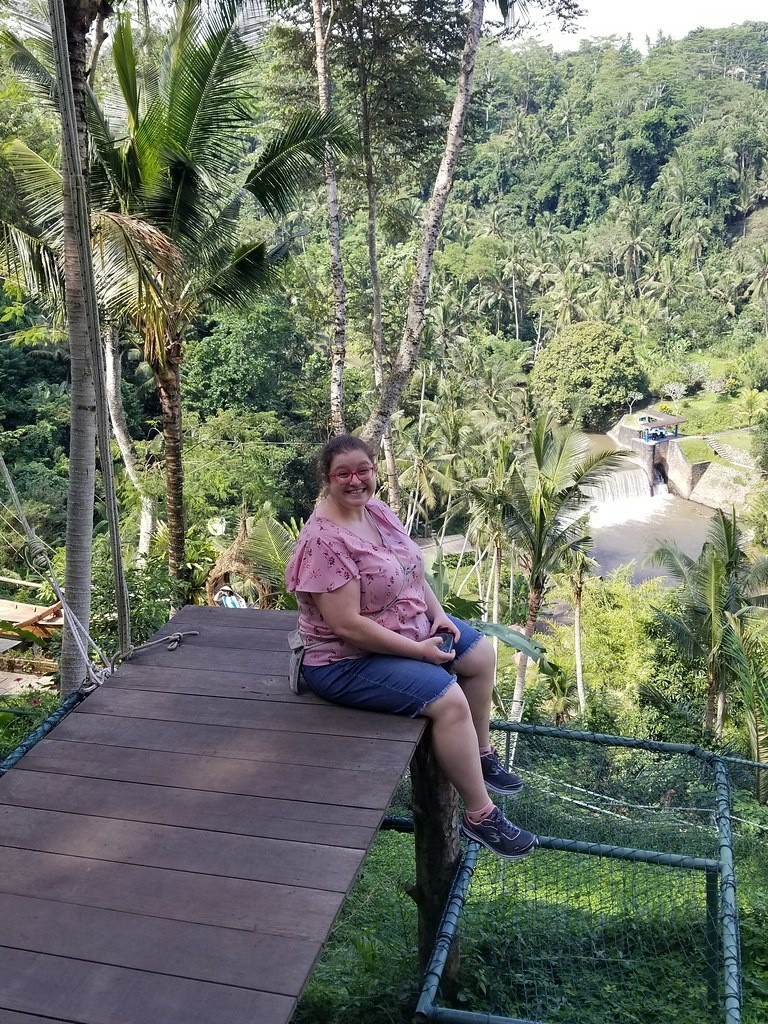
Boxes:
[328,465,378,484]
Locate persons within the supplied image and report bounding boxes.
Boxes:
[213,585,244,608]
[285,435,540,859]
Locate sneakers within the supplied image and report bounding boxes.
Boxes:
[480,748,524,795]
[460,809,539,859]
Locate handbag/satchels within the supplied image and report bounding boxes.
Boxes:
[287,629,305,693]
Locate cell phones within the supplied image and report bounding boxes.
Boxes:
[433,632,455,653]
